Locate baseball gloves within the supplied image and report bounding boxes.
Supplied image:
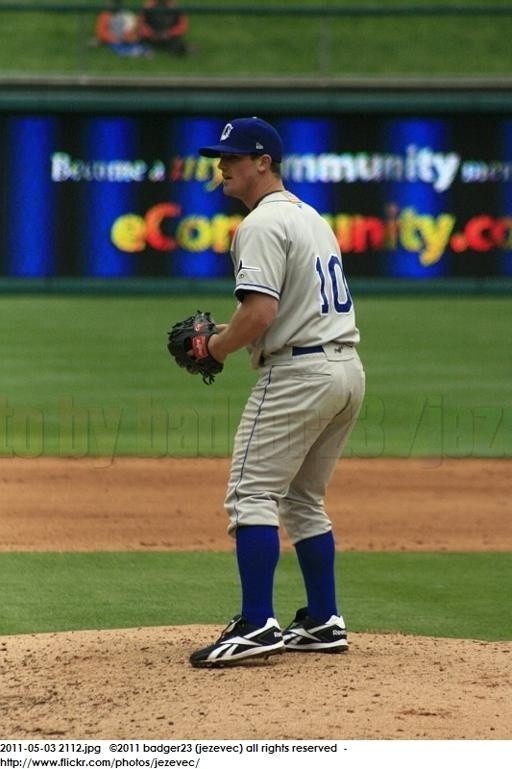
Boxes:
[166,310,224,385]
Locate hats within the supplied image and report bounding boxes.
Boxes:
[198,118,282,164]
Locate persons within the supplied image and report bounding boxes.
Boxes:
[141,0,188,55]
[95,0,146,59]
[168,116,365,668]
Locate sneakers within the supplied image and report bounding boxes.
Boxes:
[281,607,348,654]
[190,612,286,668]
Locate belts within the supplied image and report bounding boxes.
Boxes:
[257,340,325,366]
